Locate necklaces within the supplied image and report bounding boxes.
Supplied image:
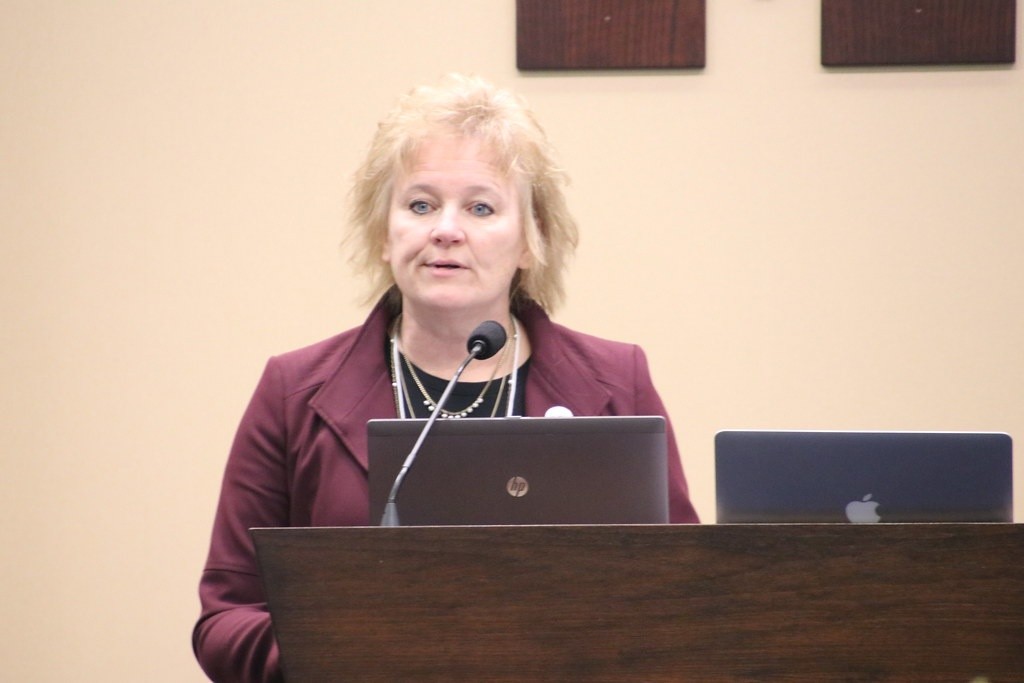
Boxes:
[390,311,520,419]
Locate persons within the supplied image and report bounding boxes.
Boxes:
[191,72,702,683]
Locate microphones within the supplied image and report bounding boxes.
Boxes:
[381,321,507,527]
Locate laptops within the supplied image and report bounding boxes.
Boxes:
[368,415,669,526]
[715,430,1014,524]
